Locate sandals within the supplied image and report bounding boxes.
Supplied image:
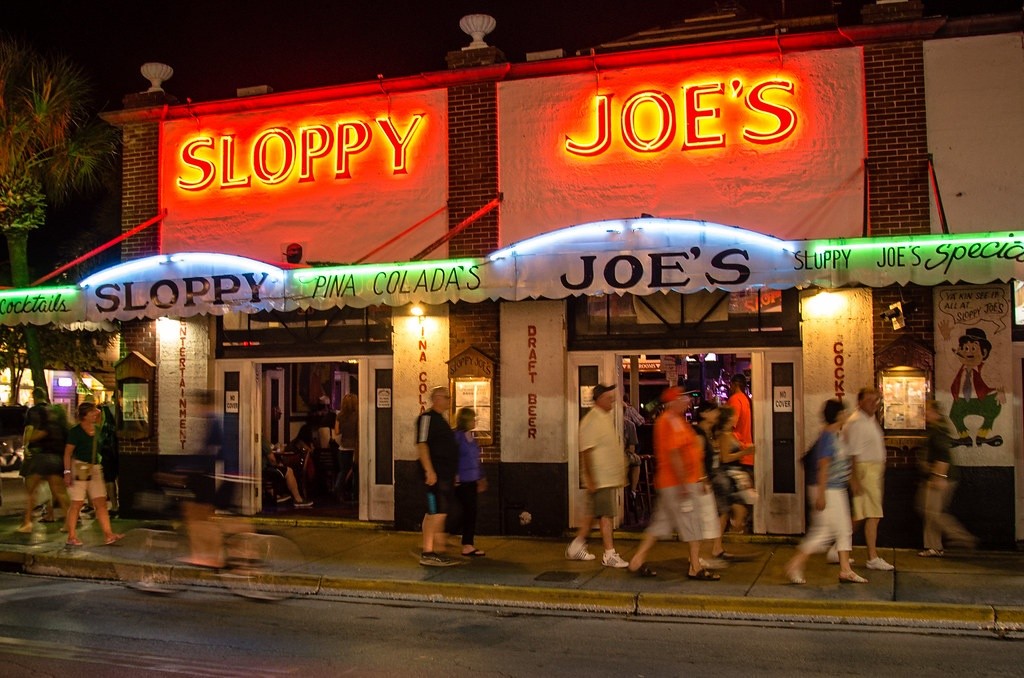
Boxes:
[918,549,944,557]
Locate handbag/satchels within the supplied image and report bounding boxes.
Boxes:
[73,463,93,482]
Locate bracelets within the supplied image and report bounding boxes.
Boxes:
[930,471,948,478]
[63,470,71,475]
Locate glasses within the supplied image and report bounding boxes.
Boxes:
[433,395,451,400]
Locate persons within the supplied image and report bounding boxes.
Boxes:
[565,384,629,569]
[414,386,464,566]
[451,408,488,557]
[779,386,977,585]
[622,401,641,498]
[63,402,125,545]
[262,393,359,507]
[13,386,81,533]
[626,373,757,581]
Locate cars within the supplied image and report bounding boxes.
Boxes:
[0,405,31,517]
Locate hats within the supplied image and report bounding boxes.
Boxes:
[593,385,615,399]
[660,386,701,404]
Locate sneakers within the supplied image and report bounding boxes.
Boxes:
[827,548,855,563]
[602,553,629,568]
[865,557,894,571]
[420,552,462,566]
[566,546,594,560]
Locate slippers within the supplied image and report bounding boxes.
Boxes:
[38,516,55,522]
[628,562,656,577]
[713,551,735,560]
[688,569,720,581]
[461,548,485,556]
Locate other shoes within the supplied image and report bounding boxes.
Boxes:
[277,495,291,502]
[839,570,868,583]
[81,506,94,512]
[15,522,32,533]
[104,533,126,544]
[780,563,807,584]
[66,536,83,546]
[294,499,314,506]
